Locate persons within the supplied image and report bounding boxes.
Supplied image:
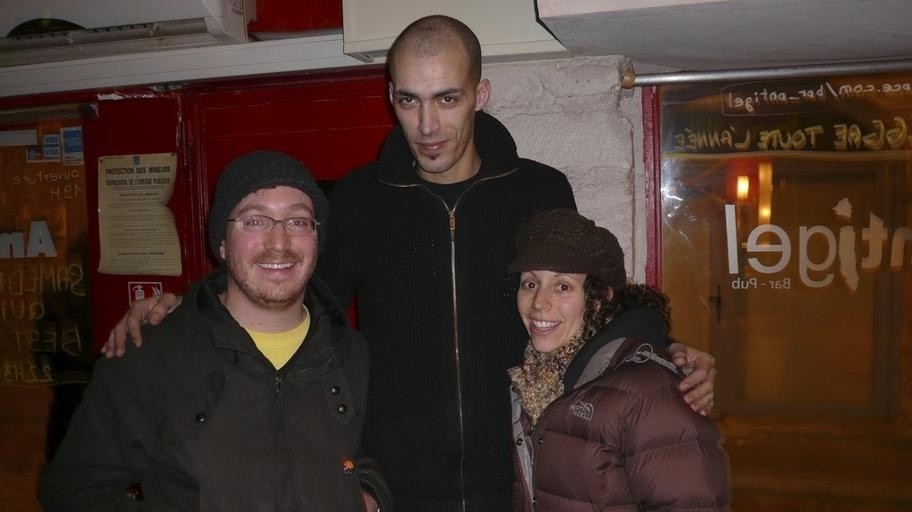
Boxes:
[505,209,733,511]
[38,150,393,512]
[98,13,718,512]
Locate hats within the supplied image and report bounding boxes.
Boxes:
[508,206,627,284]
[206,150,333,267]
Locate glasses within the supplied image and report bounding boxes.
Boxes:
[227,213,325,239]
